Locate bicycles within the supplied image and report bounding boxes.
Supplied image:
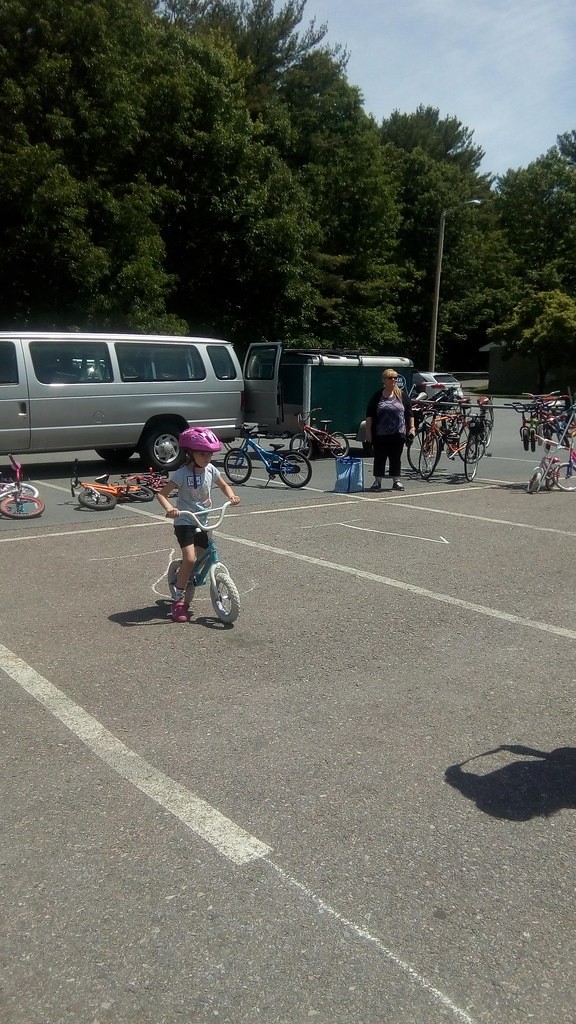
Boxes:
[70,458,178,510]
[290,408,349,462]
[165,500,241,623]
[407,383,576,494]
[224,424,312,488]
[0,453,45,518]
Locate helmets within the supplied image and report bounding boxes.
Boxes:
[179,426,222,452]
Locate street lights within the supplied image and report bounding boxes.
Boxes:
[429,199,481,372]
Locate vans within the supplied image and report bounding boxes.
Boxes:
[243,341,414,456]
[0,331,244,469]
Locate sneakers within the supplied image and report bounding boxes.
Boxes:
[393,480,405,491]
[369,480,381,493]
[171,599,189,623]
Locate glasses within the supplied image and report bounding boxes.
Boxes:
[386,377,398,381]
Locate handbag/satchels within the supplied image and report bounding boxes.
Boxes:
[335,458,365,492]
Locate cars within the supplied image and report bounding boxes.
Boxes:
[412,369,464,410]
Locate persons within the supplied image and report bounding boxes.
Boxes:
[157,427,240,623]
[365,369,415,490]
